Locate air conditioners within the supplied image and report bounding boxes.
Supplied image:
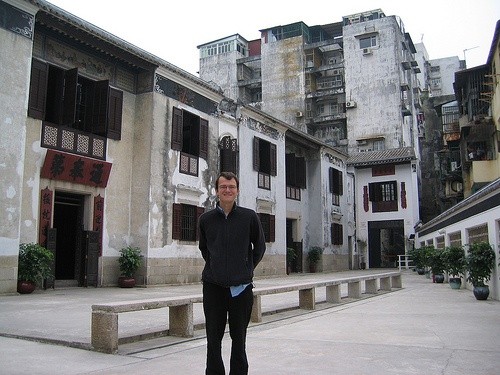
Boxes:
[362,48,372,56]
[295,111,304,117]
[433,83,439,87]
[346,100,356,108]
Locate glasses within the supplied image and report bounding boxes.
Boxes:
[217,185,237,191]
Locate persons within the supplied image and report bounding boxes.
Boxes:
[199,172,267,375]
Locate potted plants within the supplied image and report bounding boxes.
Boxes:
[308,247,322,273]
[286,248,298,275]
[18,242,55,294]
[118,245,144,288]
[408,241,496,301]
[357,239,367,269]
[381,241,405,266]
